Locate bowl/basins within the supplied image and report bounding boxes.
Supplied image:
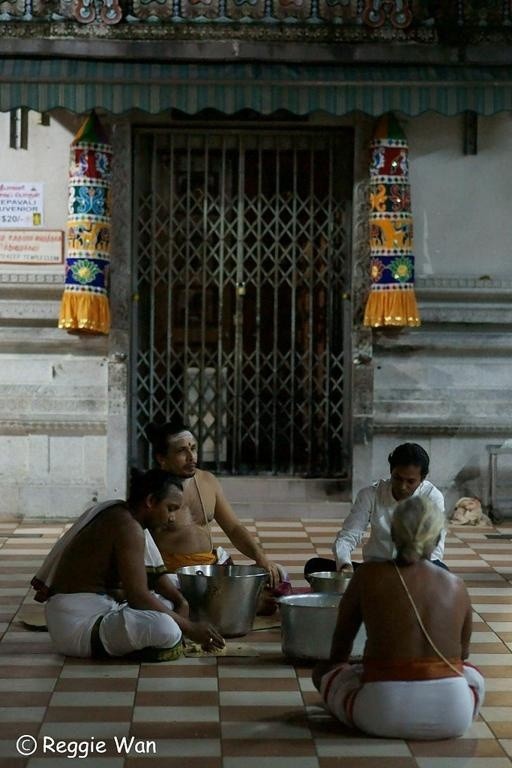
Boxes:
[310,570,354,593]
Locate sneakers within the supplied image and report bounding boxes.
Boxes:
[154,638,183,661]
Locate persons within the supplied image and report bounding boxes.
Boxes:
[303,441,451,586]
[147,418,289,619]
[310,493,489,744]
[43,463,224,664]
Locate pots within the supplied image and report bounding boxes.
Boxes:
[175,562,271,638]
[277,593,373,661]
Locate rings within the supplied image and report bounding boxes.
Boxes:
[208,637,213,643]
